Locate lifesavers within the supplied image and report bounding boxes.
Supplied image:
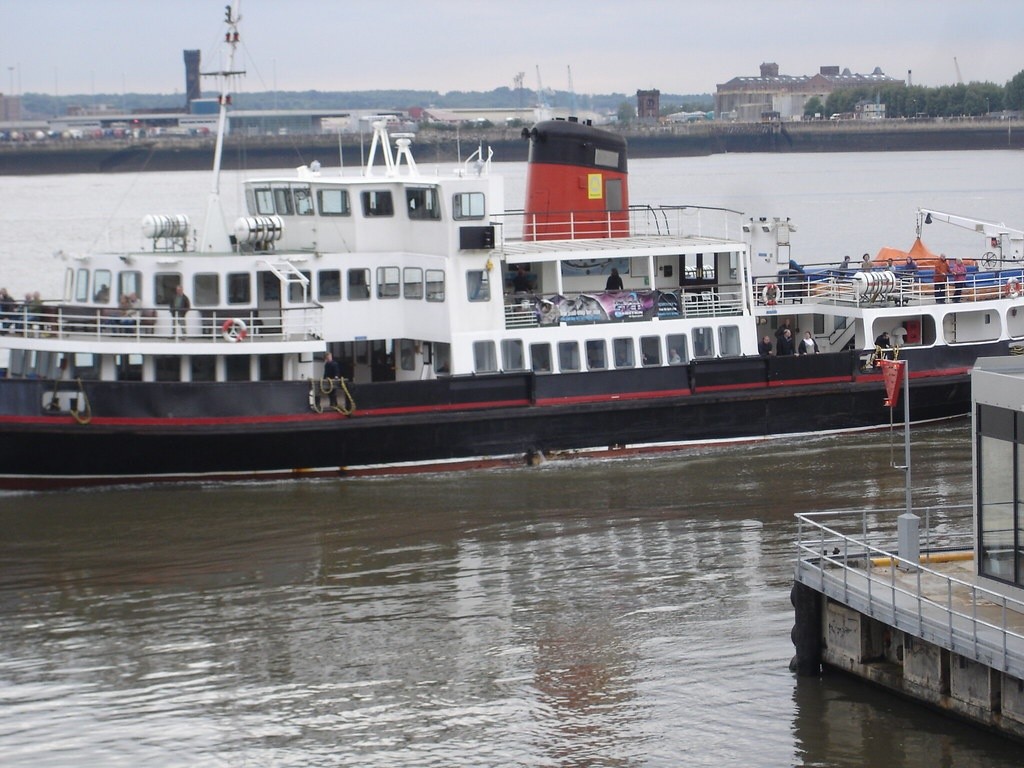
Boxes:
[762,284,780,305]
[221,318,247,343]
[1006,277,1021,299]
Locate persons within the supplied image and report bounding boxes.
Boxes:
[862,254,874,272]
[875,332,890,348]
[439,361,449,373]
[885,258,896,273]
[0,287,45,338]
[168,284,190,340]
[513,269,534,304]
[899,257,917,293]
[533,358,573,372]
[94,284,142,337]
[264,276,340,301]
[587,342,711,367]
[323,352,340,406]
[934,253,967,304]
[605,268,623,290]
[838,256,850,280]
[758,318,819,357]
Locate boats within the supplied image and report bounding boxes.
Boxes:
[0,0,1023,488]
